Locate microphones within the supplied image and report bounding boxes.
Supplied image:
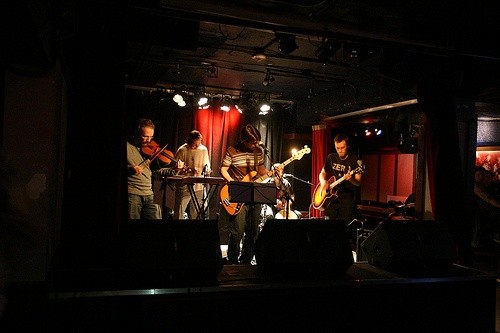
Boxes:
[259,141,269,153]
[201,164,207,175]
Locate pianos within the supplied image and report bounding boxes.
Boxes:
[161,175,225,221]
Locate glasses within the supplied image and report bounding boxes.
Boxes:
[141,135,154,139]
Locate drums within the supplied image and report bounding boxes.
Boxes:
[274,208,303,219]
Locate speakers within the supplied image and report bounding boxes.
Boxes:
[359,220,479,279]
[125,219,223,289]
[254,218,354,283]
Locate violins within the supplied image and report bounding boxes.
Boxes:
[141,140,194,174]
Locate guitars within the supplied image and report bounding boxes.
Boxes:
[219,145,311,217]
[312,164,368,211]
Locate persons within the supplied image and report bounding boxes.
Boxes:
[259,163,302,219]
[221,124,284,265]
[173,130,211,219]
[318,135,363,239]
[474,165,500,207]
[127,118,184,219]
